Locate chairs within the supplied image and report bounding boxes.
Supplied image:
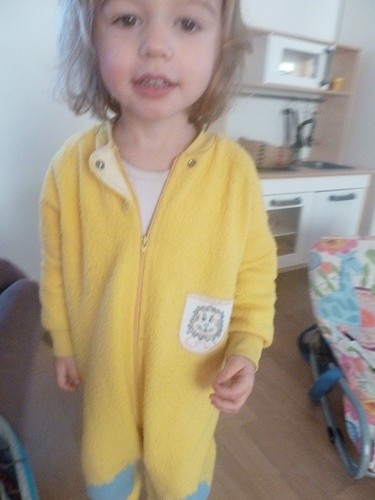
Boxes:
[299,236,375,479]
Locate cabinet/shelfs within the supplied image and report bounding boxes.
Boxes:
[215,28,374,273]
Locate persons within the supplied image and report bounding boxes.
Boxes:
[39,0,278,500]
